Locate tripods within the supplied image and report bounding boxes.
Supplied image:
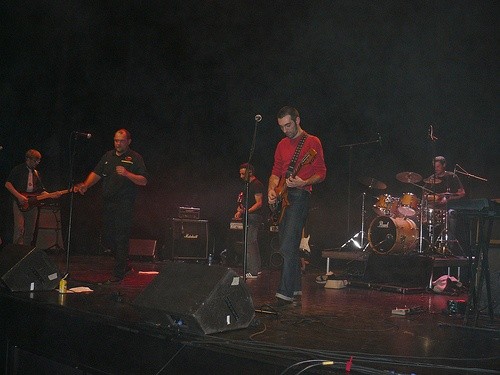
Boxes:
[340,191,372,254]
[415,142,471,260]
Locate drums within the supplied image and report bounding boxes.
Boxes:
[367,216,419,254]
[373,194,398,217]
[421,209,446,227]
[398,192,417,216]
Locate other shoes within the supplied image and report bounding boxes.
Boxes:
[257,271,262,275]
[291,286,302,295]
[275,291,293,301]
[125,263,132,273]
[111,276,122,282]
[241,273,257,278]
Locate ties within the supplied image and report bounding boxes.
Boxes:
[25,165,34,193]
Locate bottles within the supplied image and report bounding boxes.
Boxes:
[209,253,212,266]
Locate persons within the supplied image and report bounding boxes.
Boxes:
[5,148,61,245]
[234,164,266,279]
[265,105,327,308]
[424,154,466,253]
[71,129,147,277]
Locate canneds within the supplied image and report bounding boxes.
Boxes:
[208,254,214,267]
[59,279,67,293]
[448,300,456,312]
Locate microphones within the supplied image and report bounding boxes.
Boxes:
[75,131,92,138]
[254,114,262,122]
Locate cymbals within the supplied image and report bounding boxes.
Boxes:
[395,171,422,184]
[358,177,387,189]
[423,178,443,183]
[437,192,458,196]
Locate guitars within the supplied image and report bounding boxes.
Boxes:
[269,147,317,224]
[237,192,246,230]
[18,189,68,209]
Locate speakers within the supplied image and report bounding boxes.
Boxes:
[171,217,209,260]
[0,242,63,292]
[131,261,256,335]
[472,246,500,315]
